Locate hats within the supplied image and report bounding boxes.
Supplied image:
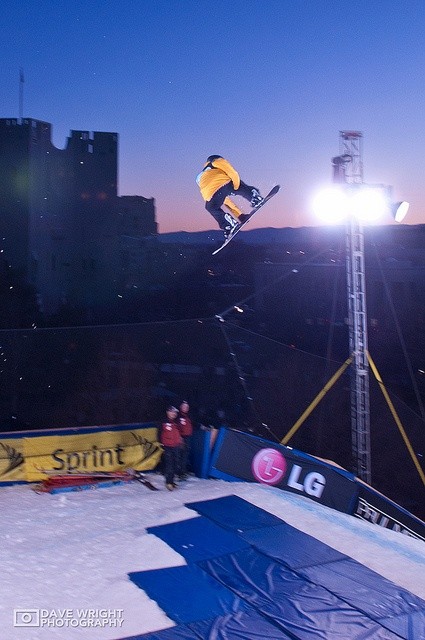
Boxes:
[167,406,178,412]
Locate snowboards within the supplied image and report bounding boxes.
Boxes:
[211,184,282,256]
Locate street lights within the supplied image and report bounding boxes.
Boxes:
[309,179,412,487]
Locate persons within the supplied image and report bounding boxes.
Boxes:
[175,400,193,482]
[195,155,264,239]
[157,406,183,492]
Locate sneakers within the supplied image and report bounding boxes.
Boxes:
[223,213,239,238]
[250,188,263,208]
[165,484,174,490]
